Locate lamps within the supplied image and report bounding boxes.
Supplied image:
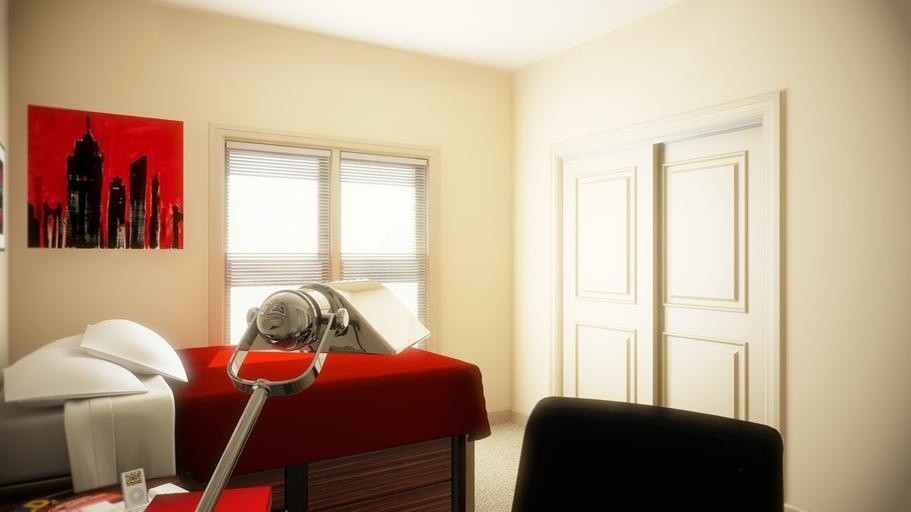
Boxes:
[190,279,431,512]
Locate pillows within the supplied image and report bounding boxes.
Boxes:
[2,334,148,406]
[81,319,188,383]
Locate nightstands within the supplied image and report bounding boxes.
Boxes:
[0,474,188,512]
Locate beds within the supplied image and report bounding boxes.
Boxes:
[1,344,492,512]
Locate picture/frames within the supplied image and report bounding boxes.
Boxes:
[0,143,5,253]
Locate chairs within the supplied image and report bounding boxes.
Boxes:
[511,397,784,512]
